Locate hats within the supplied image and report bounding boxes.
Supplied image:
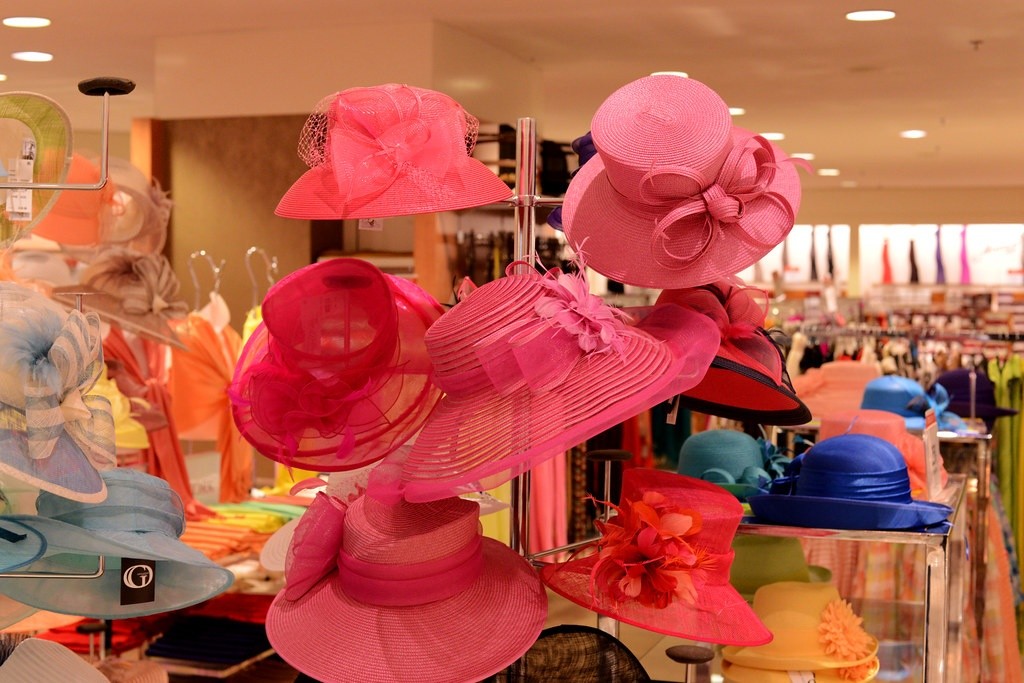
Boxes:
[0,76,1020,683]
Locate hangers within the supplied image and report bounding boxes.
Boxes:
[778,318,1023,377]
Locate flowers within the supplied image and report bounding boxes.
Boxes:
[759,453,805,497]
[840,660,880,679]
[285,477,365,600]
[86,250,190,343]
[681,280,783,387]
[469,237,657,398]
[909,382,964,429]
[298,85,476,203]
[824,601,873,660]
[737,433,787,486]
[0,300,116,467]
[583,492,705,610]
[239,359,410,464]
[637,135,812,267]
